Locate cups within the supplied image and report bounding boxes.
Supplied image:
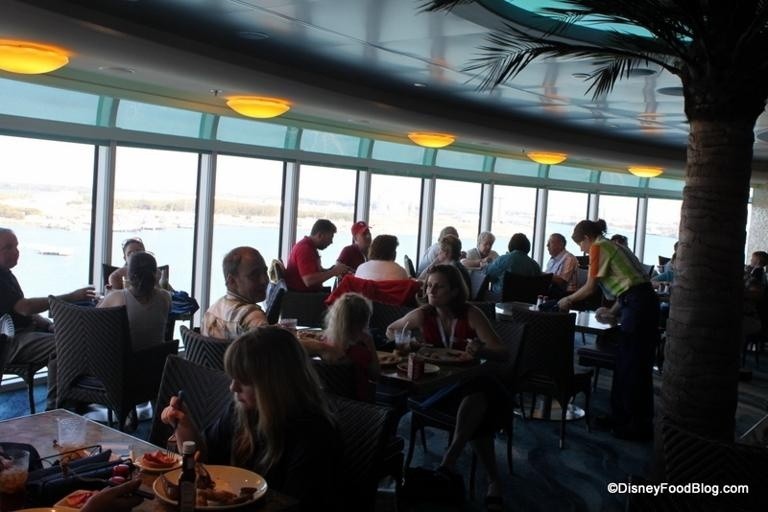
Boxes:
[395,329,412,360]
[59,417,86,446]
[2,452,28,490]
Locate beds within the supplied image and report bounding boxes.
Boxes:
[376,337,499,404]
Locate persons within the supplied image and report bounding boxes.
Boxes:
[298,291,382,374]
[557,219,660,443]
[96,250,172,353]
[460,231,500,268]
[336,220,372,283]
[160,324,343,512]
[750,250,768,288]
[285,218,347,314]
[417,226,459,274]
[386,264,503,471]
[201,245,345,366]
[0,227,97,410]
[486,233,542,303]
[418,234,473,300]
[354,234,409,282]
[545,233,580,295]
[650,252,677,291]
[108,239,146,291]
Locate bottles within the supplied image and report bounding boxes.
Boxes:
[543,296,549,304]
[536,295,544,310]
[177,440,198,512]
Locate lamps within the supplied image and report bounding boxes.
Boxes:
[407,131,458,149]
[225,96,291,119]
[0,37,75,76]
[627,166,663,178]
[526,151,567,165]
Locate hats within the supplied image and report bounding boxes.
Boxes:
[352,222,372,233]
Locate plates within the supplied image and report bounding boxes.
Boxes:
[133,455,181,471]
[154,465,268,507]
[398,364,440,373]
[417,348,472,362]
[377,350,402,363]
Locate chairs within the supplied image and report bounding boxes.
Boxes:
[147,353,231,452]
[574,341,614,414]
[408,320,529,502]
[179,326,239,371]
[47,295,158,431]
[510,304,594,452]
[266,276,287,325]
[0,361,48,415]
[330,395,396,494]
[282,291,327,328]
[103,264,168,290]
[369,300,415,333]
